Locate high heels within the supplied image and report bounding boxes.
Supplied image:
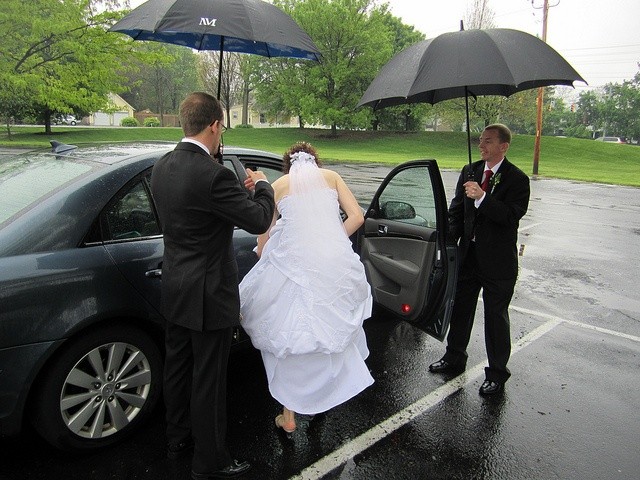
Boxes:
[275,413,296,439]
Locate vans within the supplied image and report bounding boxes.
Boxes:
[596,136,628,144]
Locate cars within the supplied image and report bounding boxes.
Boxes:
[54,113,82,126]
[0,139,462,458]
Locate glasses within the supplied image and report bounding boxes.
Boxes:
[210,119,229,134]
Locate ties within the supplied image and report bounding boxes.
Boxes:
[480,169,494,192]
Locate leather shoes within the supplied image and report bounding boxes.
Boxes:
[430,359,467,373]
[168,437,193,460]
[191,457,255,479]
[480,378,504,395]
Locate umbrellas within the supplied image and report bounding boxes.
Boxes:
[354,20,588,182]
[106,0,323,101]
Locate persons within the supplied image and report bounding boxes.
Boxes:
[151,92,274,478]
[429,123,530,394]
[238,141,374,440]
